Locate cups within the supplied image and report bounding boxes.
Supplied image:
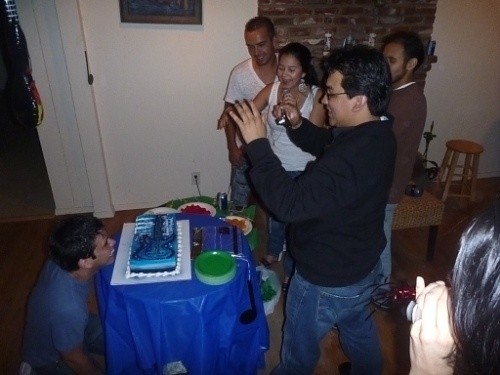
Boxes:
[343,35,354,48]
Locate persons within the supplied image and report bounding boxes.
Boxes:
[223,15,327,296]
[370,30,427,309]
[19,214,117,375]
[406,207,500,375]
[228,43,397,375]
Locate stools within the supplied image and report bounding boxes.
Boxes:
[433,138,485,210]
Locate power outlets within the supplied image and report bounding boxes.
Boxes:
[191,172,201,186]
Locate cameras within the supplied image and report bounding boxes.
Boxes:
[276,109,292,129]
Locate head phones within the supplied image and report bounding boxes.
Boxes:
[412,159,439,179]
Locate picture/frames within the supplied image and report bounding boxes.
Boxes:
[119,0,204,27]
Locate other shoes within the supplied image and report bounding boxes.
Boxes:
[370,287,392,310]
[19,361,32,375]
[338,361,351,375]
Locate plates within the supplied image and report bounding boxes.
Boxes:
[195,252,236,285]
[220,216,253,236]
[142,207,178,215]
[178,202,217,217]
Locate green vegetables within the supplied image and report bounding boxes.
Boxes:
[261,277,277,300]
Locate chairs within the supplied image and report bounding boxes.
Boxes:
[390,194,444,260]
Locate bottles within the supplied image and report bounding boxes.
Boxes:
[322,32,332,57]
[368,33,376,50]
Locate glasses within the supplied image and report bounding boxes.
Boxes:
[326,88,348,100]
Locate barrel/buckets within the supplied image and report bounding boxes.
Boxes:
[261,270,284,315]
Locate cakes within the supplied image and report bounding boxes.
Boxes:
[125,212,182,278]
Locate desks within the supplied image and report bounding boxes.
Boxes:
[96,216,268,375]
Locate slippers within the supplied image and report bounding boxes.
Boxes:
[282,282,290,295]
[260,257,278,265]
